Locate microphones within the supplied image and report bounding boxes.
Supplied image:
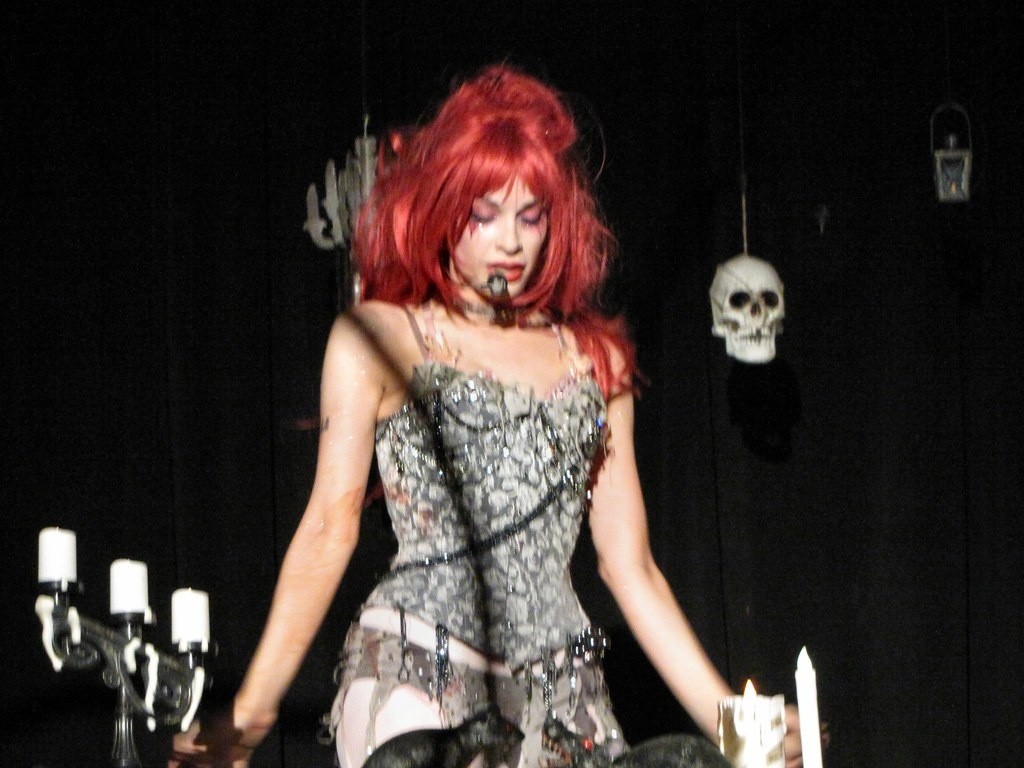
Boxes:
[485,274,509,297]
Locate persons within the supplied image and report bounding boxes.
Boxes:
[168,67,828,768]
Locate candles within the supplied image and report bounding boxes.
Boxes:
[38,525,77,583]
[794,643,823,768]
[108,558,149,614]
[170,586,210,645]
[744,678,760,767]
